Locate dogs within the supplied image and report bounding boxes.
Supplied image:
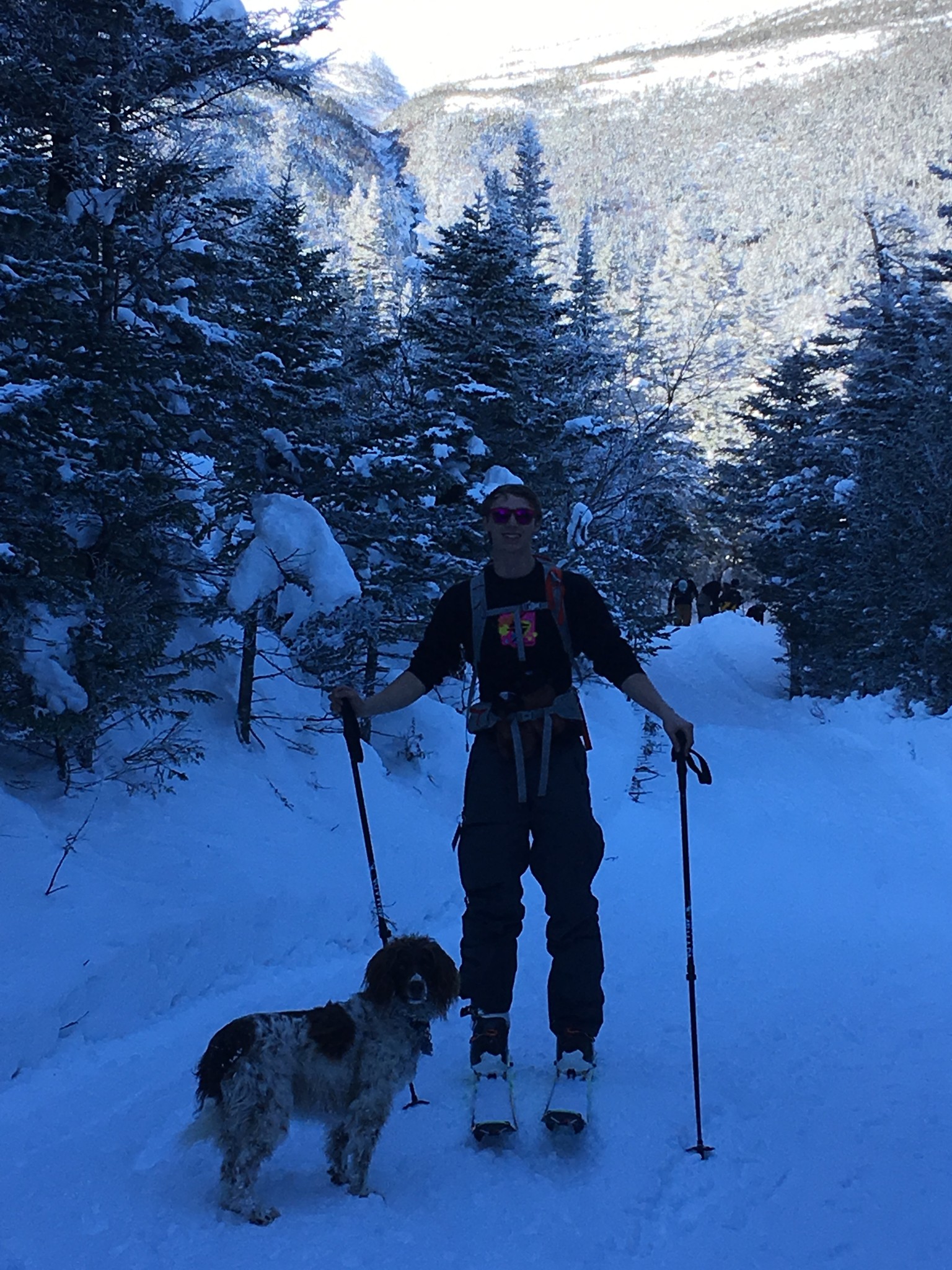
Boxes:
[747,604,767,625]
[169,933,458,1225]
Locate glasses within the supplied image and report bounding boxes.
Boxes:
[489,506,539,526]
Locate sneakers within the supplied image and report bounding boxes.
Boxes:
[461,1001,510,1076]
[553,1028,595,1078]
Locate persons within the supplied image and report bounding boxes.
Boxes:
[667,578,700,626]
[696,579,744,624]
[745,603,766,626]
[328,484,694,1142]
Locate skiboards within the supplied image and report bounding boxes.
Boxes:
[470,1034,597,1143]
